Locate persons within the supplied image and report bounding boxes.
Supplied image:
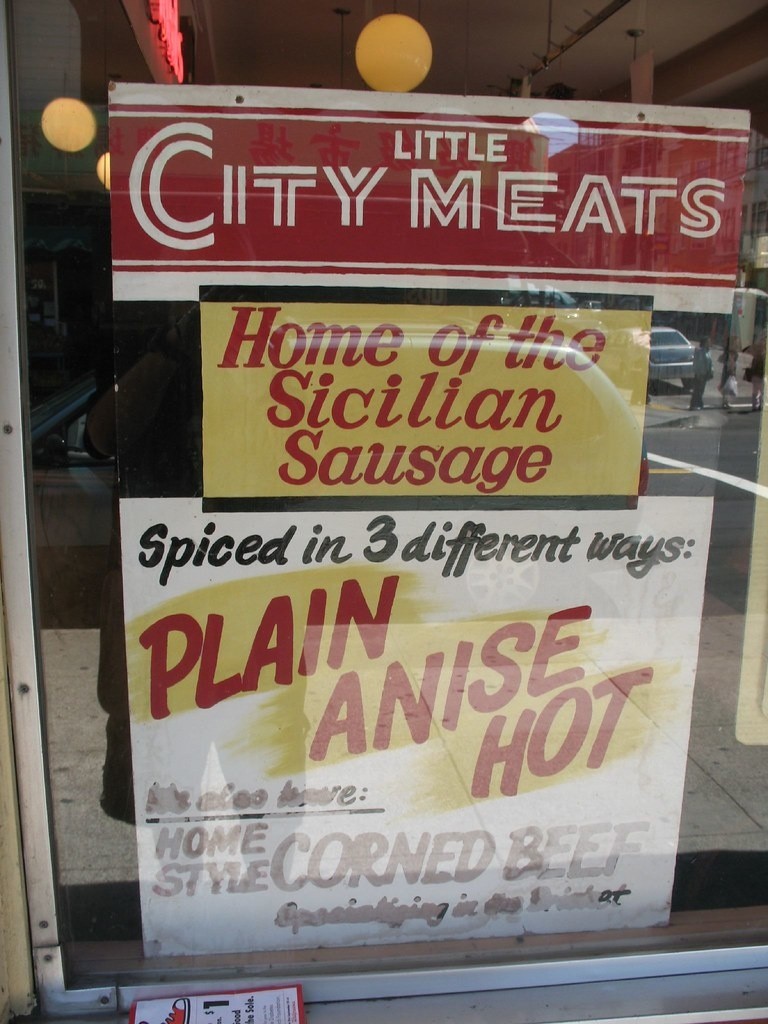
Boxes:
[82,228,310,935]
[689,337,711,411]
[718,336,766,411]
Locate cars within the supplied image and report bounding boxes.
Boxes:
[645,326,713,396]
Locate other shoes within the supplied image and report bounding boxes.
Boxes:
[690,403,704,410]
[753,405,761,411]
[723,403,731,408]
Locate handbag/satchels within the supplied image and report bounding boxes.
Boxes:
[741,367,753,382]
[718,355,727,363]
[720,375,738,397]
